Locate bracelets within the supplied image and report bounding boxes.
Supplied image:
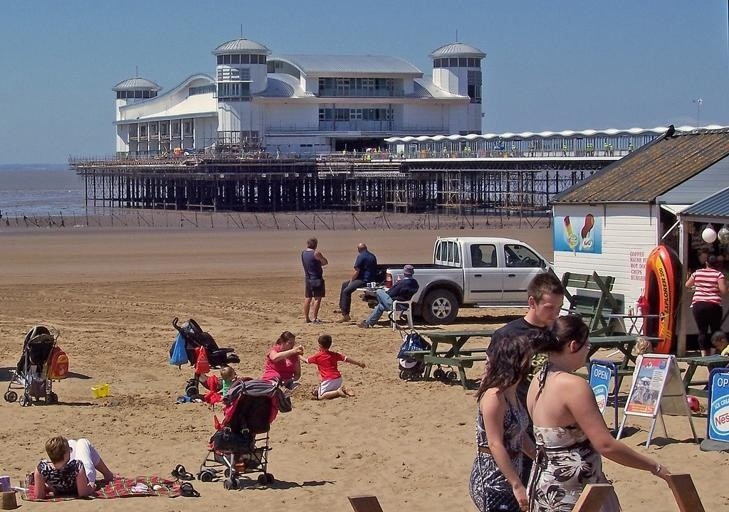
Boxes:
[654,464,661,475]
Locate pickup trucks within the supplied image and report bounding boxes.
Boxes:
[356,237,555,324]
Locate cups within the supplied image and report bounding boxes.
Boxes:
[371,282,376,288]
[367,283,371,287]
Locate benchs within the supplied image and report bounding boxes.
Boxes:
[454,356,488,361]
[402,346,491,360]
[568,366,686,399]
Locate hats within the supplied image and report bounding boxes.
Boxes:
[403,265,414,275]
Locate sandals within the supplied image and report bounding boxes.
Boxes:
[180,482,194,496]
[171,464,195,480]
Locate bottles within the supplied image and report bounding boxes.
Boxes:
[628,306,634,316]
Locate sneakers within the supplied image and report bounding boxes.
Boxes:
[304,309,369,329]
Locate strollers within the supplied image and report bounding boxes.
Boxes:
[197,377,294,489]
[396,328,457,384]
[4,325,61,406]
[170,318,241,396]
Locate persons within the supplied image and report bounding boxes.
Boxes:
[220,367,235,396]
[298,335,365,400]
[485,274,564,488]
[261,331,304,387]
[685,255,728,357]
[711,331,729,357]
[301,238,329,323]
[358,265,419,328]
[333,243,377,324]
[469,335,538,512]
[525,315,672,512]
[34,436,112,499]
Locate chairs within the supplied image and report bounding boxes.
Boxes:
[387,288,421,332]
[471,246,488,266]
[491,248,508,267]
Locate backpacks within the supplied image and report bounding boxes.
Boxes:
[46,347,70,380]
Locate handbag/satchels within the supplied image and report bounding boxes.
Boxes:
[194,346,210,374]
[170,332,188,365]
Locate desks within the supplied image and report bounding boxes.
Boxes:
[589,335,665,394]
[682,354,729,394]
[418,329,497,390]
[606,313,659,336]
